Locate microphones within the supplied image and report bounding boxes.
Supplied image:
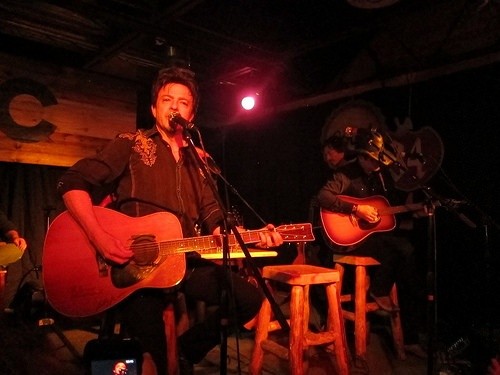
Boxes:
[168,111,197,131]
[344,127,377,135]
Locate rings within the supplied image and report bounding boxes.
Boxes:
[266,245,271,249]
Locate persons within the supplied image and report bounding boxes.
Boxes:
[57,68,283,375]
[316,131,452,358]
[0,211,27,250]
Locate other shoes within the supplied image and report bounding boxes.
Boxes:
[176,339,193,375]
[405,344,428,358]
[368,288,400,312]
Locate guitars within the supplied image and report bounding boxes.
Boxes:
[319,195,457,252]
[43,207,316,318]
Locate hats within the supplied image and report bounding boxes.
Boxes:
[352,128,392,165]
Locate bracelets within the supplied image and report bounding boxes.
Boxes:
[352,204,358,214]
[415,211,421,218]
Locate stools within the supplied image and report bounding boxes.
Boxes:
[247,264,349,375]
[322,254,404,361]
